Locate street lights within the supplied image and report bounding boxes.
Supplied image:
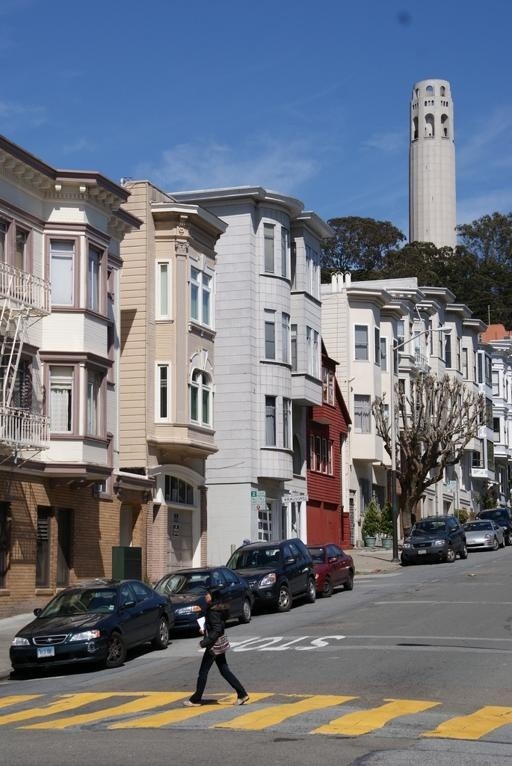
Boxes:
[390,327,454,562]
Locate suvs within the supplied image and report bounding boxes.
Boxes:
[225,538,317,614]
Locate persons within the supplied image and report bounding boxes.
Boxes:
[182,587,249,707]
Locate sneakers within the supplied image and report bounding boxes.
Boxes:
[183,701,201,707]
[233,695,249,706]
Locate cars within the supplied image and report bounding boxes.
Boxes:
[401,508,512,566]
[9,578,175,680]
[154,565,253,636]
[304,543,355,598]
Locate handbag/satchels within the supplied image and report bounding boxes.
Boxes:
[208,636,230,655]
